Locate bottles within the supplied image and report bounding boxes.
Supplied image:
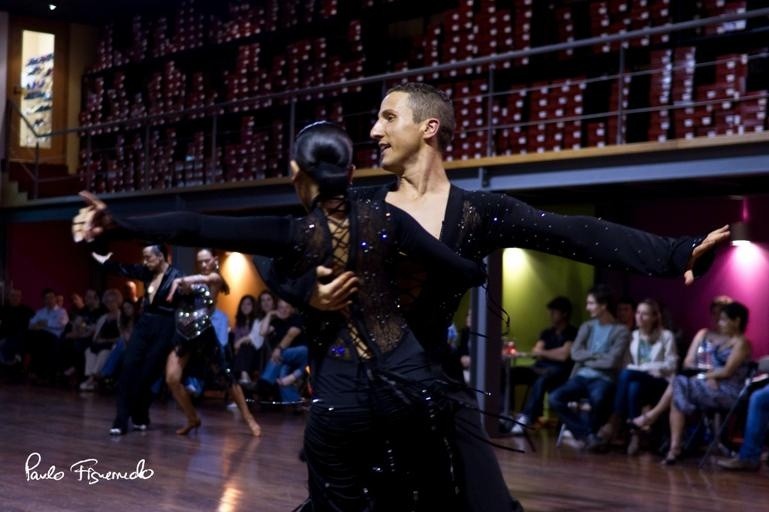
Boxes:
[505,340,516,358]
[706,339,716,369]
[696,343,707,370]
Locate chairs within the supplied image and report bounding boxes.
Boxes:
[685,362,759,473]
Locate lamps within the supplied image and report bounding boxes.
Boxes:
[729,194,752,246]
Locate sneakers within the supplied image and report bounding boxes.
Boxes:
[512,415,527,433]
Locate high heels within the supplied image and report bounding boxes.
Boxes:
[176,417,201,435]
[246,416,264,437]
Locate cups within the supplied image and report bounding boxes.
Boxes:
[501,337,509,356]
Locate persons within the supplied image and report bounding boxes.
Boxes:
[79,81,729,511]
[0,233,769,472]
[72,123,486,511]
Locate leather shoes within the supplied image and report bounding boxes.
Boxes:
[629,434,640,455]
[666,446,684,463]
[597,422,617,439]
[710,455,760,469]
[627,413,653,430]
[583,433,597,452]
